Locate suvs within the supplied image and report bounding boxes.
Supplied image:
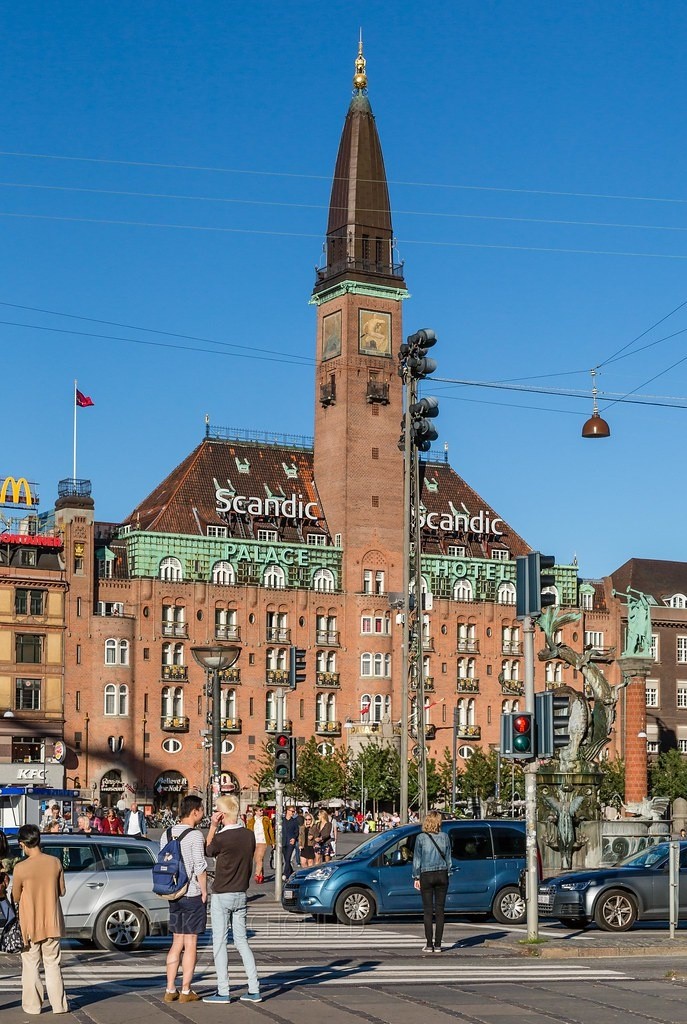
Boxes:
[281,820,545,925]
[0,831,173,952]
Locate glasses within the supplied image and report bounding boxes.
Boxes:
[288,810,295,813]
[304,818,312,821]
[19,845,23,849]
[257,808,263,812]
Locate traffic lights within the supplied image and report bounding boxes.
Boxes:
[542,692,570,759]
[274,733,293,783]
[508,712,535,760]
[290,646,307,691]
[526,550,557,618]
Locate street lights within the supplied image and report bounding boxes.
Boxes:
[395,328,441,826]
[189,644,243,832]
[346,760,364,816]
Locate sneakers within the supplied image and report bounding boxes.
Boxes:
[239,992,263,1002]
[202,992,231,1003]
[434,946,441,952]
[422,946,433,952]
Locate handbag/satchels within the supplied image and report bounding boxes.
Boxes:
[0,890,25,954]
[111,830,117,834]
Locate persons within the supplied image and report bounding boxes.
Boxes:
[201,794,263,1004]
[10,824,71,1015]
[43,793,146,870]
[1,829,16,923]
[159,795,209,1003]
[240,802,419,883]
[412,810,452,953]
[159,805,173,824]
[677,829,687,841]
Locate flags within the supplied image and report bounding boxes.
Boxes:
[75,389,94,407]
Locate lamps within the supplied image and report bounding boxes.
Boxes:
[4,708,15,718]
[580,368,611,438]
[637,729,648,738]
[343,719,354,729]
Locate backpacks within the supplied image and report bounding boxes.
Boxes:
[153,826,198,900]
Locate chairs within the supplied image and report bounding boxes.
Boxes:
[453,838,471,858]
[83,858,93,866]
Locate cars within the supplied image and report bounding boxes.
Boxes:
[538,840,687,933]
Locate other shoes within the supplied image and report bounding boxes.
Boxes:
[282,875,286,882]
[164,988,180,1002]
[178,990,200,1004]
[254,875,265,884]
